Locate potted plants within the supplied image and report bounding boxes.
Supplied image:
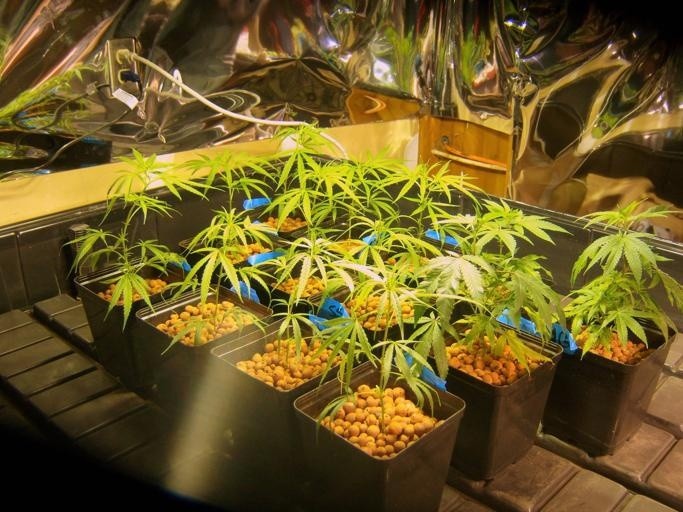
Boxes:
[244,125,336,240]
[179,152,289,287]
[245,166,359,313]
[133,208,275,420]
[541,201,682,456]
[291,147,382,255]
[209,240,371,469]
[413,251,564,482]
[74,149,203,389]
[293,248,488,512]
[307,161,438,356]
